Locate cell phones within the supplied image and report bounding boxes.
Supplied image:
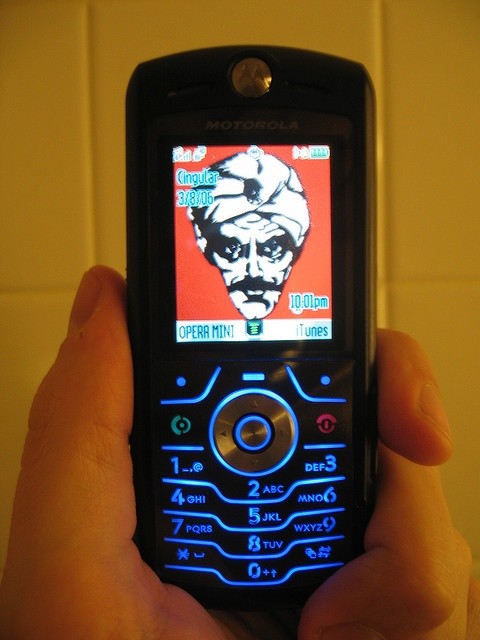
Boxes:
[124,43,377,619]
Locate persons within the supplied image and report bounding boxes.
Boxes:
[187,146,310,319]
[3,264,479,638]
